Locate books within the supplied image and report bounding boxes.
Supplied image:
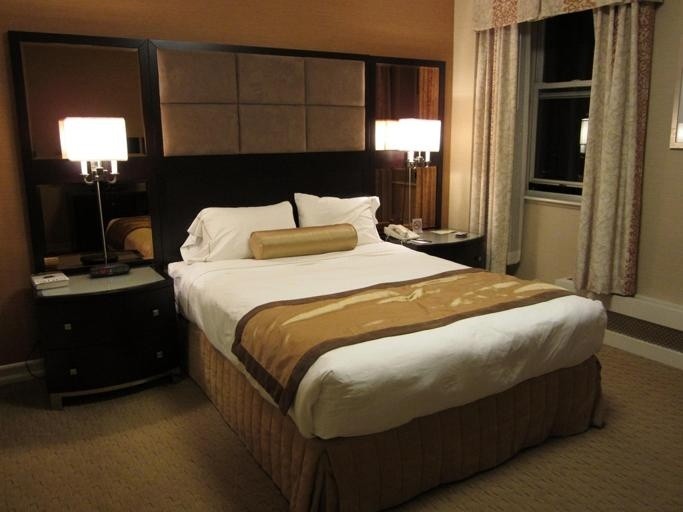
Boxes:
[28,271,70,291]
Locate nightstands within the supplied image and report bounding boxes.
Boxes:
[33,261,183,411]
[386,226,487,270]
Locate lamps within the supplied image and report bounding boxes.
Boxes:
[56,114,130,276]
[377,119,442,226]
[401,117,443,228]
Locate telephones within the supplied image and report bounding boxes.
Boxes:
[384,223,420,241]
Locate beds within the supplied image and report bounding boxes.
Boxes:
[160,152,609,512]
[106,212,154,263]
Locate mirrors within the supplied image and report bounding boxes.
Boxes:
[375,64,441,224]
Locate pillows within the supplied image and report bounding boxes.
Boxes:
[294,193,382,246]
[180,201,297,262]
[248,223,358,260]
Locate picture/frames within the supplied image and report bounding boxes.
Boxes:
[670,56,683,150]
